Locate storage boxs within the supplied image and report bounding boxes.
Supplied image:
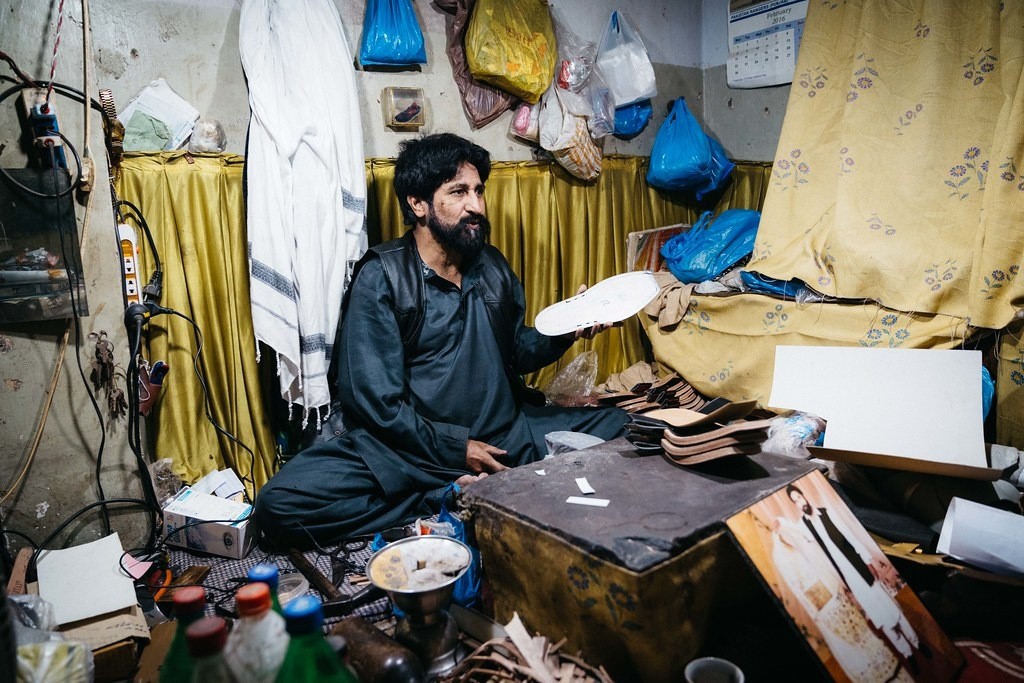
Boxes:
[463,430,831,682]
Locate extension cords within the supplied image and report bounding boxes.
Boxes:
[21,87,72,172]
[117,223,144,307]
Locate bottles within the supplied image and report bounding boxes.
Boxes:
[155,562,358,682]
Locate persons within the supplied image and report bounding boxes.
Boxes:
[253,133,632,553]
[785,484,938,683]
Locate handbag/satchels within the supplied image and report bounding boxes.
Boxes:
[596,10,657,109]
[532,68,607,183]
[666,99,733,207]
[661,203,764,287]
[435,0,517,126]
[601,10,655,139]
[645,95,711,190]
[357,1,429,68]
[547,2,598,91]
[468,1,556,101]
[508,93,542,144]
